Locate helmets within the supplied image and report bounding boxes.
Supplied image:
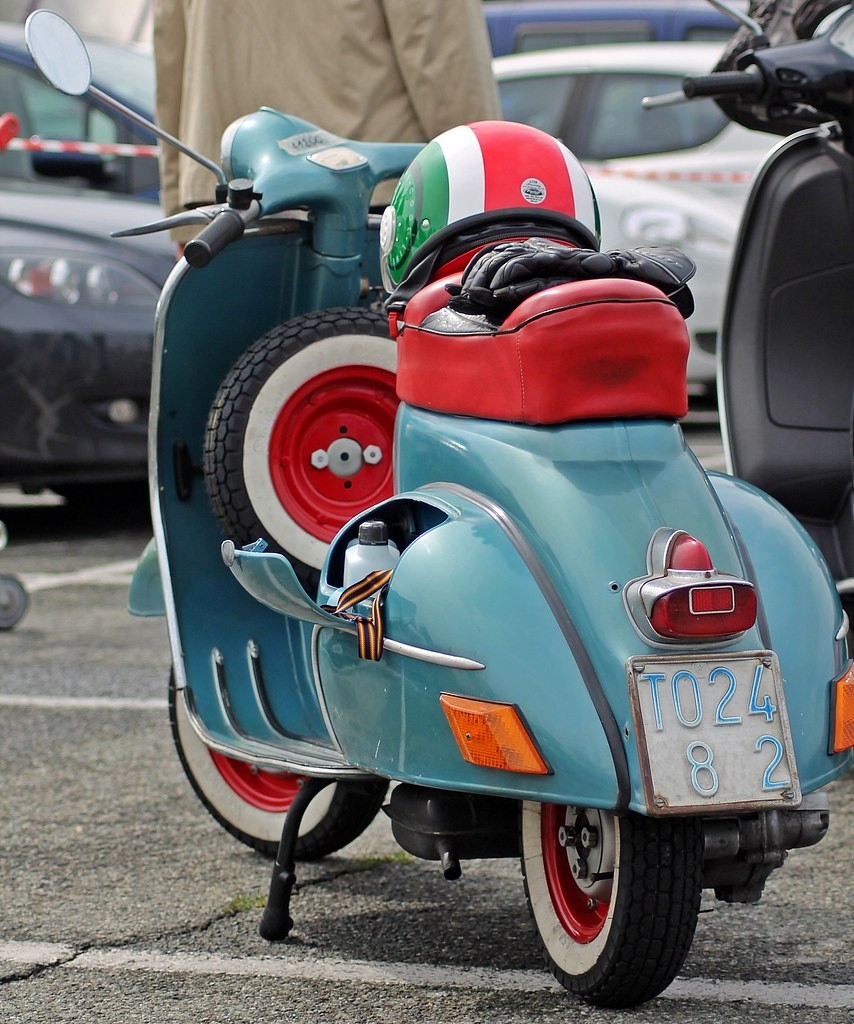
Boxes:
[379,119,602,294]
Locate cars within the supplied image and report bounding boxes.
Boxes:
[0,22,169,208]
[486,9,742,57]
[0,180,184,503]
[475,40,806,406]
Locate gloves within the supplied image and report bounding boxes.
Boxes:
[445,234,697,327]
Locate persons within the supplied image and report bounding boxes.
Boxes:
[149,2,504,265]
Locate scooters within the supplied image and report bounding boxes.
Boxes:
[640,2,853,659]
[22,8,852,1005]
